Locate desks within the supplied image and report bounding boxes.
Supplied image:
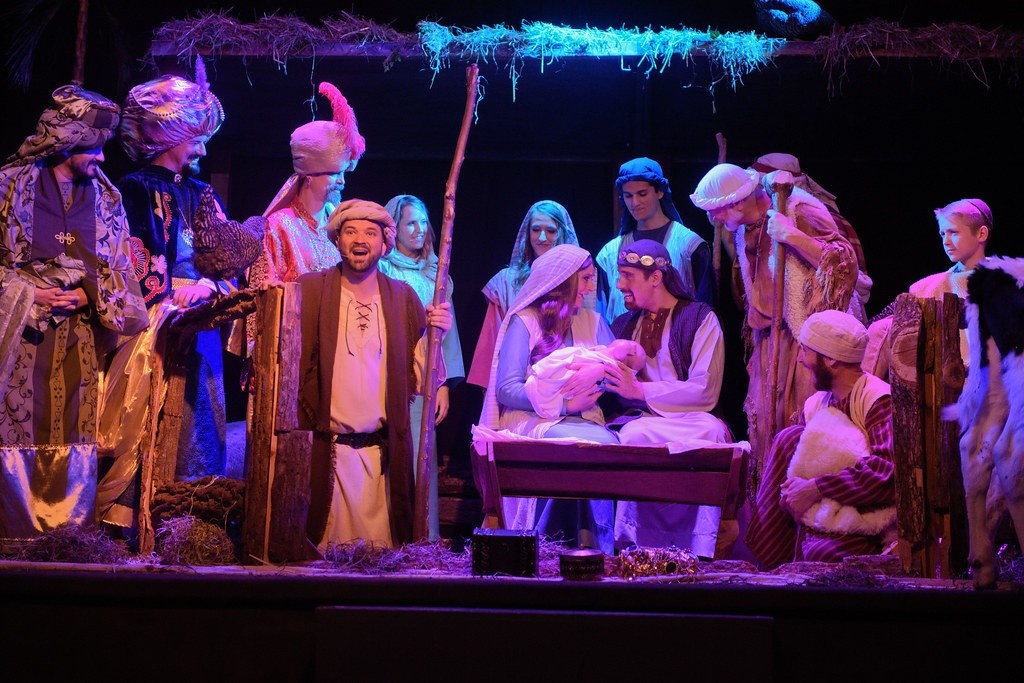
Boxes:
[468,424,752,563]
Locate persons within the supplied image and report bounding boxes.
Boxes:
[246,197,451,565]
[117,55,268,486]
[686,162,862,508]
[465,199,607,526]
[939,264,1024,591]
[527,337,647,420]
[260,79,366,567]
[0,81,150,447]
[594,157,712,326]
[470,241,647,550]
[741,308,898,565]
[905,196,1024,305]
[603,239,738,562]
[383,194,466,548]
[706,153,865,364]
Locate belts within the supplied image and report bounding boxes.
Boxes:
[327,434,384,448]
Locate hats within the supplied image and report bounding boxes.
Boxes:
[1,86,122,173]
[798,310,869,364]
[327,198,397,254]
[225,80,365,355]
[617,158,685,235]
[119,55,224,161]
[689,163,760,210]
[967,197,993,223]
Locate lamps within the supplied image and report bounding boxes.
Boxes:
[560,549,606,579]
[471,527,541,577]
